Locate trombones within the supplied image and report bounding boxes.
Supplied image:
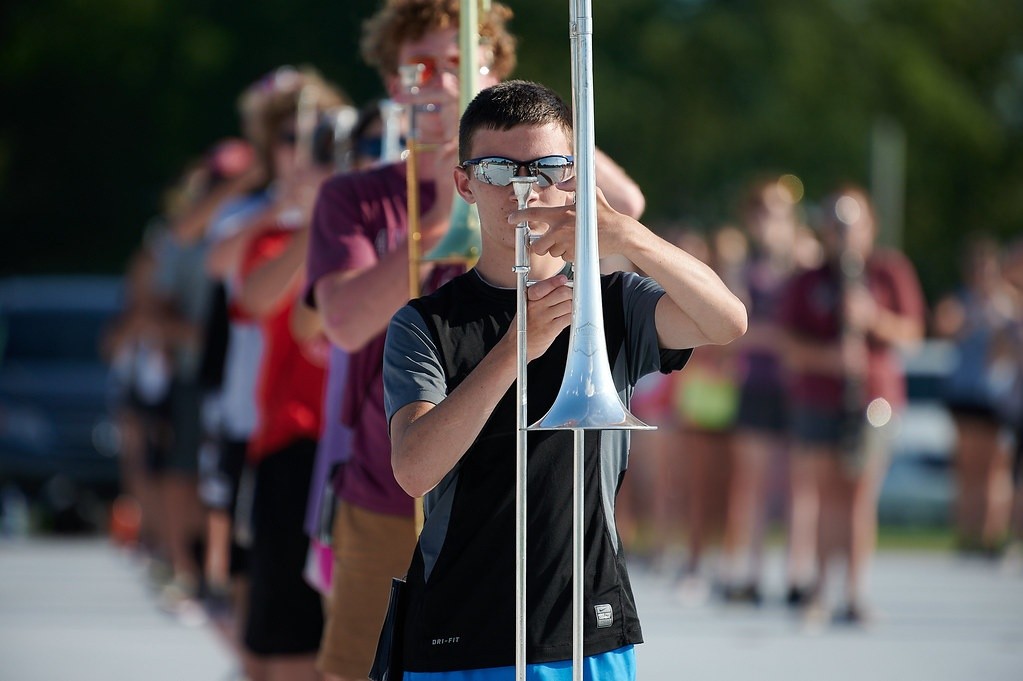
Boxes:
[510,0,658,681]
[401,0,480,549]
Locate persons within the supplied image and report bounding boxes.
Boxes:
[302,0,645,680]
[616,178,923,626]
[101,61,385,681]
[383,78,748,681]
[936,236,1023,562]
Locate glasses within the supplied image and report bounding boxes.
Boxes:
[397,53,495,90]
[461,154,574,188]
